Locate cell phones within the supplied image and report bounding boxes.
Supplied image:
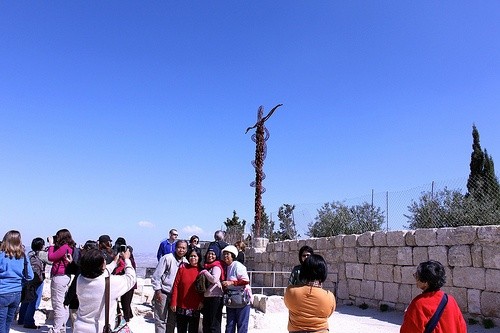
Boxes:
[120,245,127,254]
[66,249,69,257]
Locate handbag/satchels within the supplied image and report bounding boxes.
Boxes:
[103,315,133,333]
[196,274,206,292]
[223,285,248,308]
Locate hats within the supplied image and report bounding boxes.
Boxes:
[222,245,238,258]
[207,245,220,258]
[97,235,112,242]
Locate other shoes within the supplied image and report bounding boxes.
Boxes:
[26,323,41,329]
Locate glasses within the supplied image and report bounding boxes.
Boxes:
[301,254,310,257]
[413,273,419,279]
[172,234,178,236]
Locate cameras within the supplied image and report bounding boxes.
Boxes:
[47,235,57,243]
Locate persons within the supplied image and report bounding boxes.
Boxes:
[73,246,137,333]
[49,228,76,333]
[222,245,252,333]
[186,235,202,266]
[151,240,188,333]
[235,241,245,264]
[204,230,229,307]
[287,245,314,285]
[179,245,224,333]
[19,237,50,328]
[157,229,180,261]
[400,260,467,333]
[115,237,137,322]
[171,247,203,333]
[284,254,336,333]
[35,235,126,324]
[0,230,34,333]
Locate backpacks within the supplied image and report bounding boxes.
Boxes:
[24,250,43,283]
